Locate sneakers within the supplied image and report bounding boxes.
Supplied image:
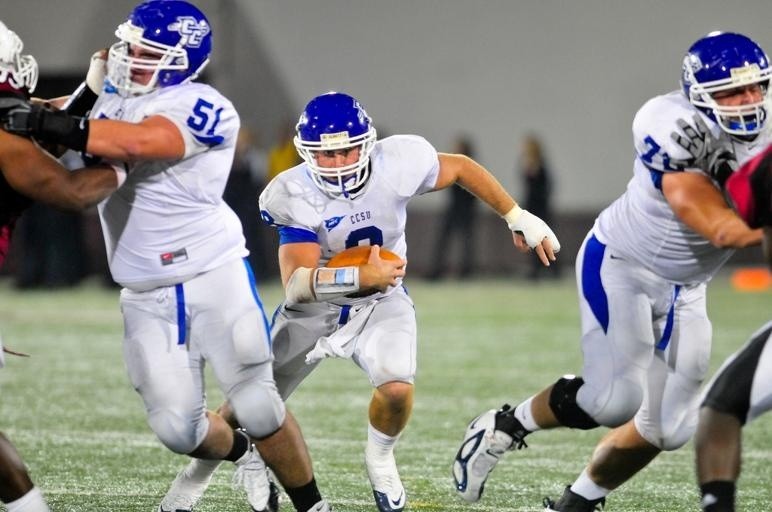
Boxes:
[450,408,533,503]
[364,441,407,512]
[237,444,279,512]
[156,473,206,511]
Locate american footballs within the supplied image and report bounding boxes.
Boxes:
[325,246,404,297]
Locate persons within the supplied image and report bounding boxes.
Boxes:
[0,23,141,512]
[521,132,560,279]
[231,122,297,277]
[1,0,332,512]
[694,139,772,511]
[424,131,479,282]
[450,28,772,512]
[156,89,561,512]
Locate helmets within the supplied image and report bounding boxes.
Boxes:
[679,30,771,141]
[104,1,214,98]
[0,16,40,101]
[291,89,380,204]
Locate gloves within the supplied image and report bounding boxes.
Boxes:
[0,92,90,154]
